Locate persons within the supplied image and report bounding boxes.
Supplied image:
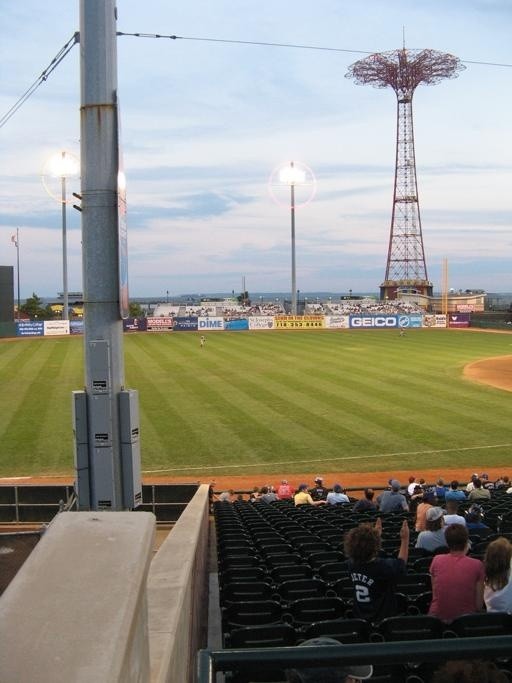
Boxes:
[199,335,206,349]
[399,324,407,336]
[208,477,350,514]
[343,473,512,622]
[159,300,429,316]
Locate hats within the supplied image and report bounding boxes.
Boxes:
[299,484,308,490]
[314,478,321,484]
[389,480,401,490]
[334,484,341,492]
[426,507,448,521]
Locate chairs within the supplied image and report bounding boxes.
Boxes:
[211,482,511,682]
